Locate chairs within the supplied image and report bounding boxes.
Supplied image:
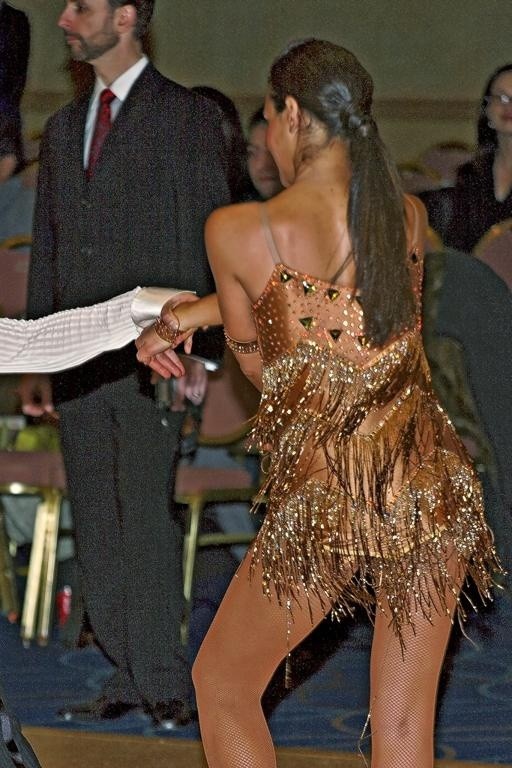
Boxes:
[0,230,259,651]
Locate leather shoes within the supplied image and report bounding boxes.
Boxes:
[55,695,133,721]
[152,697,186,730]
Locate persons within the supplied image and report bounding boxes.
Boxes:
[195,86,497,555]
[19,2,234,730]
[136,38,506,768]
[0,288,208,768]
[451,63,512,249]
[1,4,31,177]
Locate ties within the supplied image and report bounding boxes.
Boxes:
[86,88,116,184]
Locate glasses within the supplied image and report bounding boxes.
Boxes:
[486,92,511,105]
